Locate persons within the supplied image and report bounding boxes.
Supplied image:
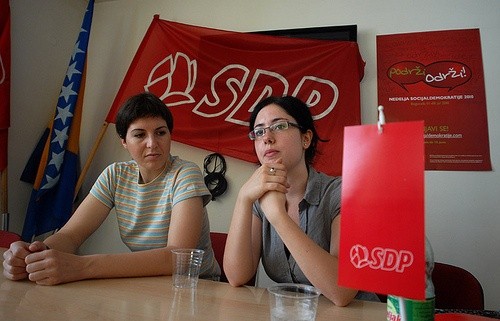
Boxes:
[223,95,381,307]
[2,92,222,286]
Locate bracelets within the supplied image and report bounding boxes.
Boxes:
[45,244,50,250]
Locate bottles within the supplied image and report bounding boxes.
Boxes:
[386,235,436,321]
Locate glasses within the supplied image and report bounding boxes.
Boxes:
[248,120,304,141]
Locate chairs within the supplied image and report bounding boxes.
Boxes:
[376,260,486,312]
[210,232,260,289]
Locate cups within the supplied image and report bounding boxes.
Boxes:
[267,283,322,321]
[170,249,205,289]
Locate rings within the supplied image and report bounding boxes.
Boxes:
[270,168,276,176]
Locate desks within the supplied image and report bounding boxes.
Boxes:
[0,245,492,321]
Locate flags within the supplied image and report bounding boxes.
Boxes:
[0,0,11,176]
[104,19,366,177]
[18,0,95,244]
